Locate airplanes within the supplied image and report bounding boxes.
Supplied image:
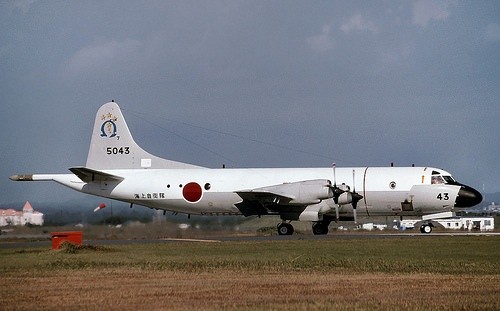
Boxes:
[10,101,483,237]
